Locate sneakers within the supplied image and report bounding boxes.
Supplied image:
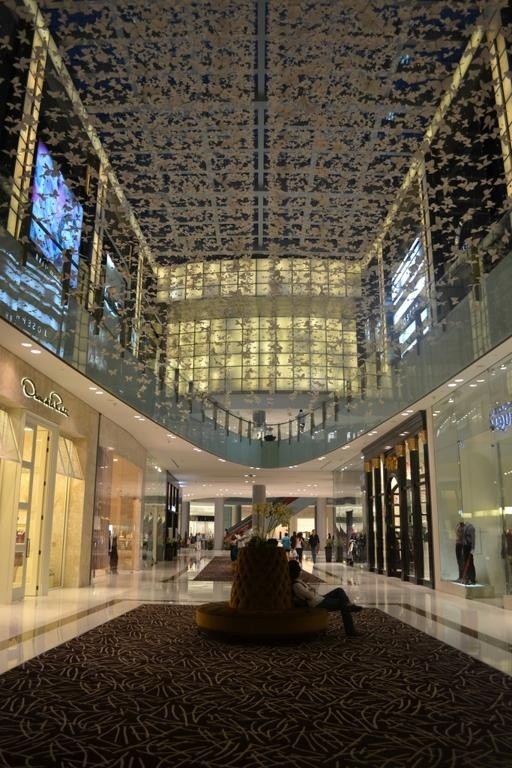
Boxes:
[348,631,364,636]
[344,605,362,612]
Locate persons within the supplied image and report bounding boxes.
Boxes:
[461,521,476,585]
[175,528,363,566]
[451,521,465,583]
[108,530,119,575]
[295,408,306,431]
[289,561,364,639]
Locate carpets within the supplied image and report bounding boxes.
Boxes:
[194,555,326,583]
[1,602,510,766]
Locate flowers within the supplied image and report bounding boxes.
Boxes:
[251,502,292,544]
